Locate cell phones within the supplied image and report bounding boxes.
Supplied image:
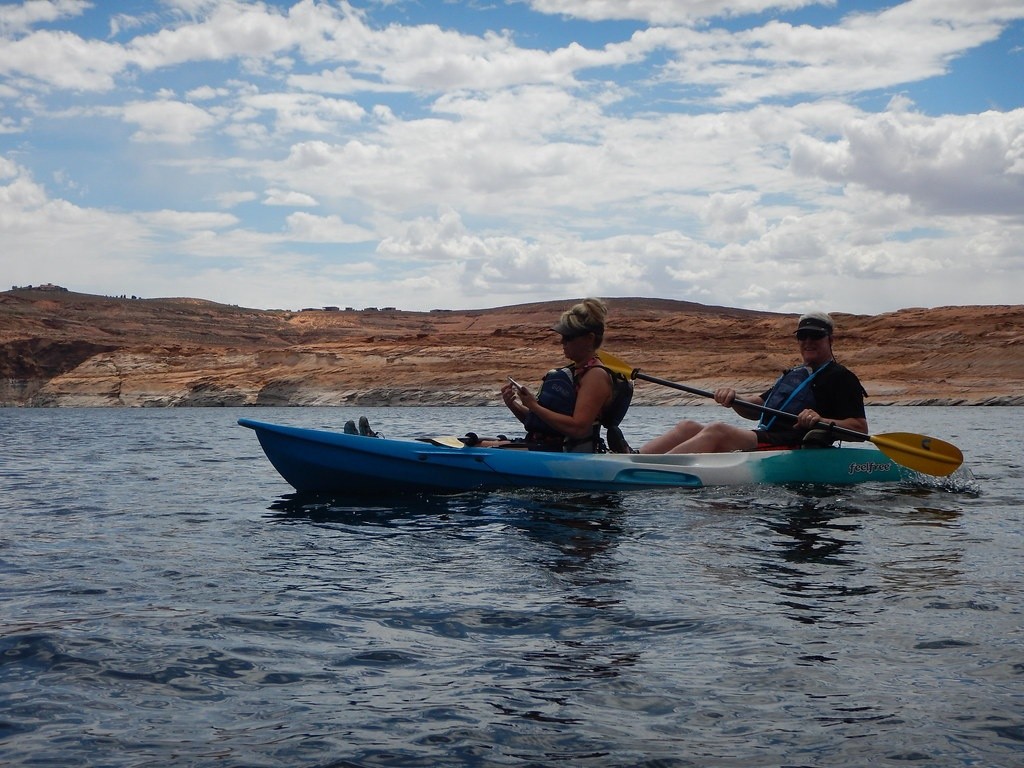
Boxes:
[507,377,523,393]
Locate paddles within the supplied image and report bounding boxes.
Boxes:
[596,349,965,478]
[414,432,512,449]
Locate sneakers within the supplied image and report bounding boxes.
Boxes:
[593,437,615,455]
[359,416,385,440]
[607,426,641,454]
[344,420,358,435]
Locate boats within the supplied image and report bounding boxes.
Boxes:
[237,419,963,492]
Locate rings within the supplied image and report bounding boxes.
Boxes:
[809,415,812,418]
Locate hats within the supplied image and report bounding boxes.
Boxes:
[793,317,834,335]
[548,324,602,338]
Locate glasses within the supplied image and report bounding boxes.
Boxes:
[562,333,589,342]
[796,330,828,341]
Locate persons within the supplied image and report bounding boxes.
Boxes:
[343,299,612,454]
[608,313,868,454]
